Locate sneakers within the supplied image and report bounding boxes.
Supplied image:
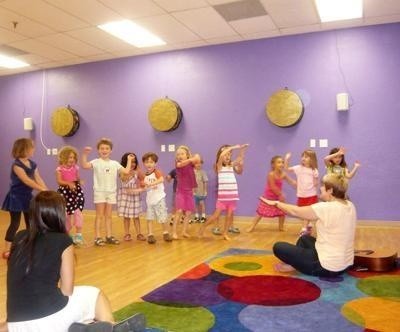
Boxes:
[3,250,10,259]
[212,226,221,235]
[227,226,241,233]
[166,216,206,224]
[124,233,173,243]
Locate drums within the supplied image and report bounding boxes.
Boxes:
[49,107,79,136]
[149,98,182,132]
[266,89,304,128]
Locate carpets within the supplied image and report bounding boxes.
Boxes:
[109,248,400,332]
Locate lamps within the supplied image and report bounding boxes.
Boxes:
[24,118,33,130]
[336,93,349,111]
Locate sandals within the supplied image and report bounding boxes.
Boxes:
[106,236,121,245]
[94,237,107,247]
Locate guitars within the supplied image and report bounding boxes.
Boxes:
[354,246,400,272]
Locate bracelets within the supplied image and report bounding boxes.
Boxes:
[275,201,279,206]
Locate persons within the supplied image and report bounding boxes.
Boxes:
[259,174,357,277]
[7,191,115,332]
[323,148,360,179]
[284,150,319,230]
[1,139,249,261]
[246,156,297,233]
